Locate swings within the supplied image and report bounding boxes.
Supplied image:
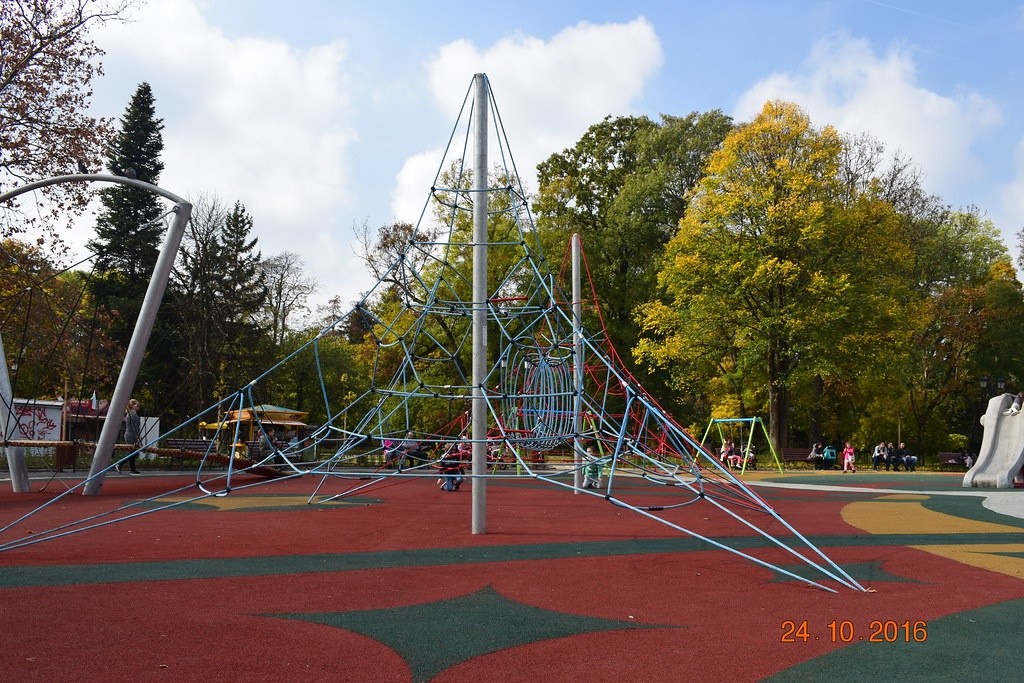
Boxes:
[730,421,753,467]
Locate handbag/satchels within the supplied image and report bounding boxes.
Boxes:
[848,454,855,463]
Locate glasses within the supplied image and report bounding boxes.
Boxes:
[134,405,140,407]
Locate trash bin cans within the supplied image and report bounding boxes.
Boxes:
[56,444,76,472]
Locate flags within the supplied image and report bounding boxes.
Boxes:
[91,390,96,409]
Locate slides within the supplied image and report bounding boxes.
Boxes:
[963,390,1024,489]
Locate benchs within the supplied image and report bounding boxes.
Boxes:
[938,452,968,472]
[780,447,833,470]
[165,438,218,470]
[715,448,740,458]
[244,441,303,470]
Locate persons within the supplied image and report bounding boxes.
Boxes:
[260,427,286,471]
[403,432,428,469]
[238,430,244,444]
[807,442,824,471]
[1002,391,1024,416]
[719,438,756,468]
[458,436,472,470]
[872,441,917,471]
[287,431,299,448]
[385,430,404,468]
[436,443,467,491]
[256,429,264,442]
[962,446,974,469]
[843,442,856,473]
[582,446,603,488]
[114,398,141,475]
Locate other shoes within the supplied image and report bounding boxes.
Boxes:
[852,470,855,473]
[1003,412,1010,415]
[441,481,446,489]
[453,484,459,490]
[401,464,404,469]
[129,471,141,476]
[116,465,122,473]
[1011,411,1019,415]
[388,465,392,469]
[731,465,735,467]
[736,464,742,468]
[843,471,847,473]
